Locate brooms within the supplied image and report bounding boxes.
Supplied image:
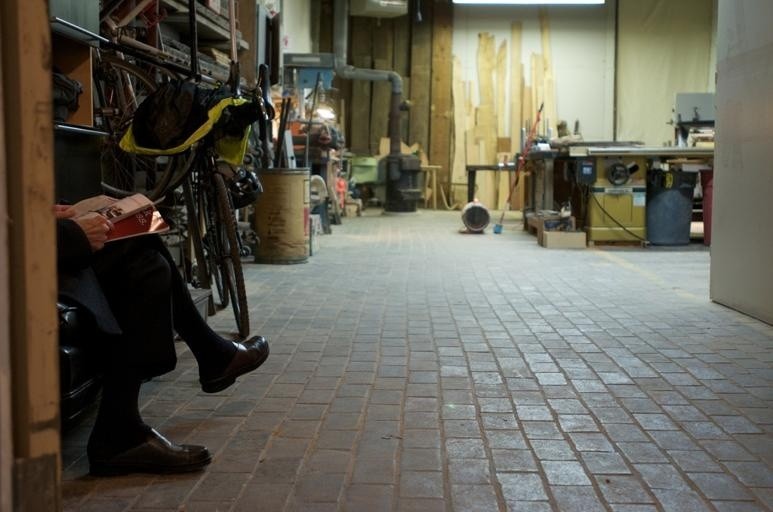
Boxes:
[494,102,544,234]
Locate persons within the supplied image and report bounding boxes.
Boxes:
[55,205,269,477]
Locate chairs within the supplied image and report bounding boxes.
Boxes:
[57,296,110,435]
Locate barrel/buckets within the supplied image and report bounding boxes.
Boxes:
[251,166,310,264]
[462,202,490,232]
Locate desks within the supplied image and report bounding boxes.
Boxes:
[467,164,530,229]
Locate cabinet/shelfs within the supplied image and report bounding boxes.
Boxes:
[49,1,112,205]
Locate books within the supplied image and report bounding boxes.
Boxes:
[63,193,170,243]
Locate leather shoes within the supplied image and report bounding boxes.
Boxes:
[85,428,213,477]
[198,335,270,394]
